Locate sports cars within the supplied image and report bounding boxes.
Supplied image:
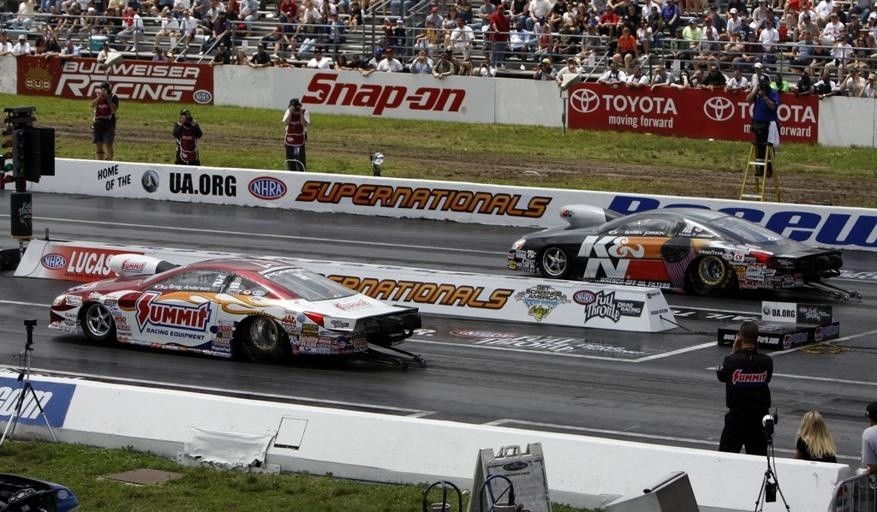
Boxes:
[502,198,869,306]
[44,253,433,376]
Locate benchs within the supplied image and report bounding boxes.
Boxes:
[0,1,877,92]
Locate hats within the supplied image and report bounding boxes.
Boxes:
[705,16,712,21]
[730,7,738,13]
[543,58,550,63]
[688,18,694,23]
[18,35,25,39]
[755,62,762,68]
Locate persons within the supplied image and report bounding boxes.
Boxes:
[282,100,310,172]
[747,75,781,177]
[173,110,203,166]
[795,412,838,462]
[90,83,119,161]
[0,0,877,98]
[716,322,773,456]
[856,403,877,474]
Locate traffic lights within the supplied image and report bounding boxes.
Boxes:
[18,128,55,183]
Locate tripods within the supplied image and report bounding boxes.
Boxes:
[0,348,58,447]
[754,445,791,512]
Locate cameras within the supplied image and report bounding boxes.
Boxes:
[760,81,767,89]
[185,110,193,122]
[105,81,112,94]
[294,99,302,112]
